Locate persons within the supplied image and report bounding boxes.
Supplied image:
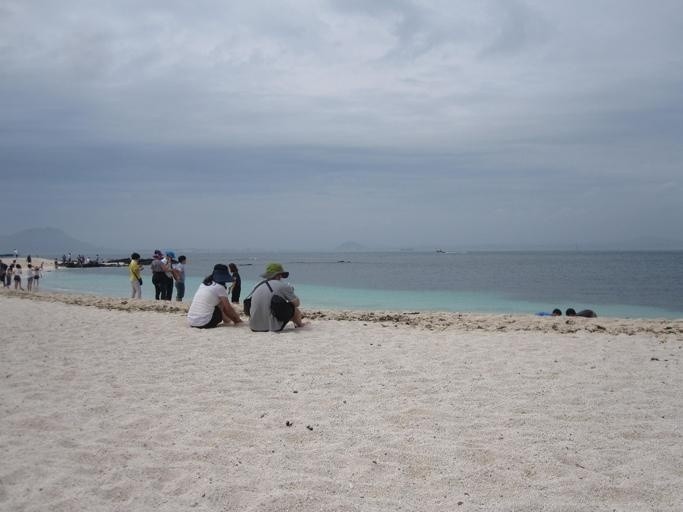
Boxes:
[128,252,144,299]
[248,263,306,332]
[151,249,187,301]
[187,263,243,329]
[534,307,562,316]
[0,248,100,292]
[227,263,241,303]
[565,307,597,318]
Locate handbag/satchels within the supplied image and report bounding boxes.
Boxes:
[271,295,294,321]
[139,278,142,285]
[244,299,251,315]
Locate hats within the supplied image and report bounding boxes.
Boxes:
[166,252,174,258]
[153,251,161,257]
[213,264,233,282]
[259,264,289,279]
